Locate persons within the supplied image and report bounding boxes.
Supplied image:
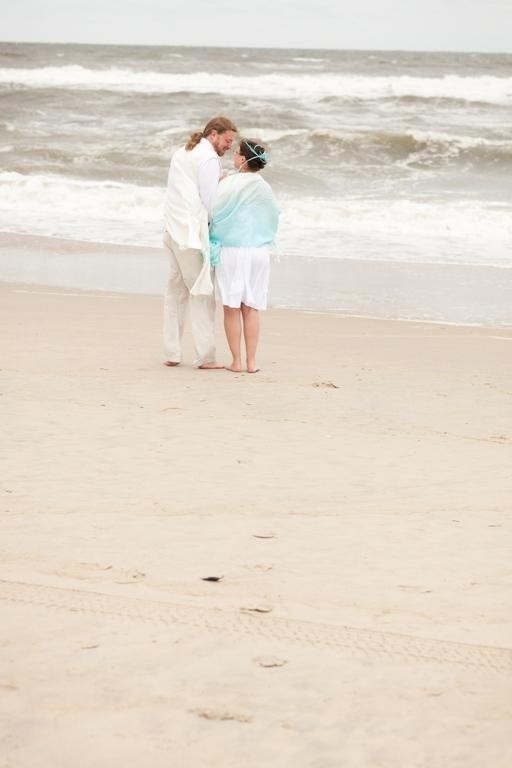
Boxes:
[210,137,277,373]
[161,117,238,369]
